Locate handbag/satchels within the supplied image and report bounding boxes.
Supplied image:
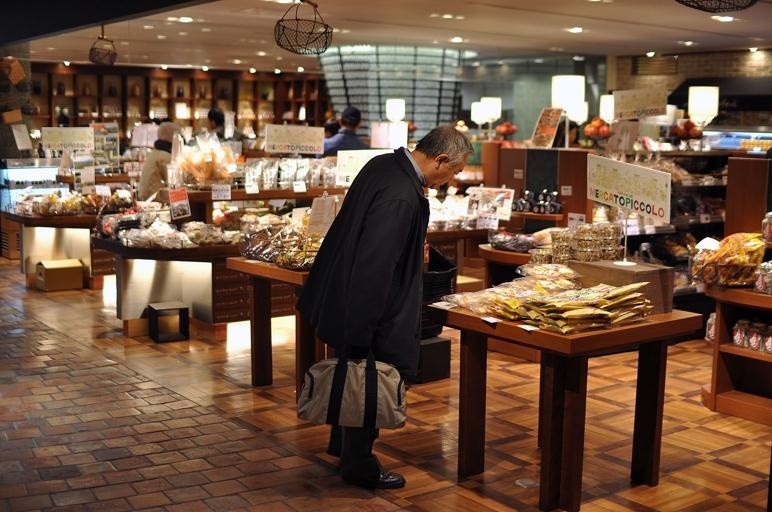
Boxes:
[295,321,409,430]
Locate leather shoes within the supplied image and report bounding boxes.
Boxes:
[339,455,406,489]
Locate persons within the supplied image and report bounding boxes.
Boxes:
[323,118,341,136]
[293,125,476,490]
[555,121,579,147]
[139,121,185,202]
[198,108,249,142]
[316,107,370,159]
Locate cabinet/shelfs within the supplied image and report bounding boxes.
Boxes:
[707,155,771,436]
[11,62,328,151]
[0,152,474,360]
[475,139,717,330]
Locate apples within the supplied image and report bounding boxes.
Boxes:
[673,119,702,139]
[583,117,612,137]
[495,121,517,135]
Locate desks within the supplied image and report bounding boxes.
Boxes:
[227,253,482,415]
[427,298,701,510]
